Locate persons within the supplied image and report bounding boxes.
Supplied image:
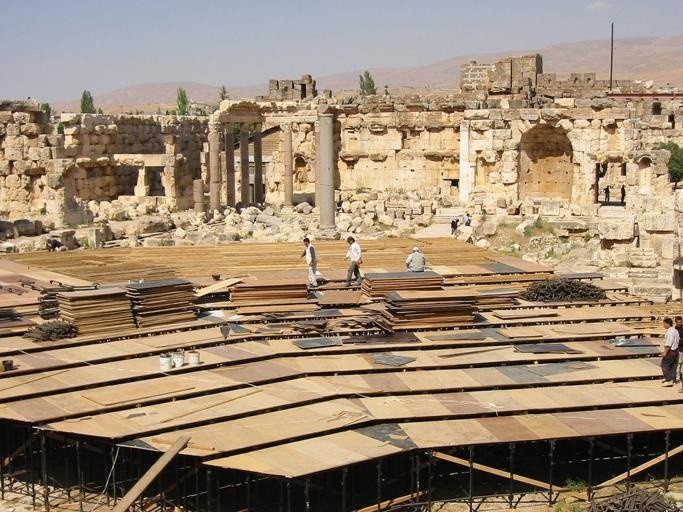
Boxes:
[451,217,460,234]
[659,317,680,386]
[464,213,471,226]
[674,315,683,394]
[343,236,363,287]
[299,238,318,288]
[46,238,67,253]
[621,184,626,205]
[405,246,426,273]
[602,185,611,204]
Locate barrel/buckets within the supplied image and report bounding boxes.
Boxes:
[188,350,200,364]
[160,357,171,371]
[173,352,183,368]
